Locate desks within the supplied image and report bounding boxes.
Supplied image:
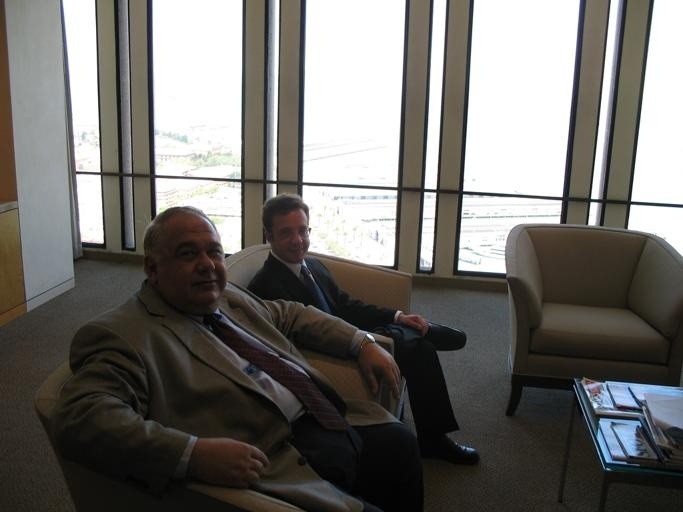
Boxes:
[556,371,682,512]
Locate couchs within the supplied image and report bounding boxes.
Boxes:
[32,233,424,512]
[497,222,682,419]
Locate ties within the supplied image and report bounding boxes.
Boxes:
[205,313,347,432]
[302,266,332,314]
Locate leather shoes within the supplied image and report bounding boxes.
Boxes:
[421,440,480,464]
[427,322,466,351]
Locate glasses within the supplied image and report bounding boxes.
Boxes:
[276,227,310,241]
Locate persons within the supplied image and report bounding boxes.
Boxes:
[48,206,424,510]
[246,192,478,466]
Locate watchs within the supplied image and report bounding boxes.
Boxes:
[360,333,374,351]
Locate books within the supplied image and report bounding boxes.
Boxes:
[581,376,682,470]
